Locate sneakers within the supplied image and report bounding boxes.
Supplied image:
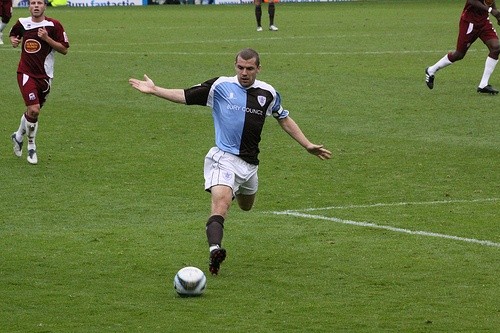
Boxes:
[425,67,433,89]
[477,85,499,95]
[26,147,38,164]
[11,132,23,157]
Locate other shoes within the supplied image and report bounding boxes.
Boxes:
[257,26,263,31]
[208,248,226,275]
[0,38,4,44]
[269,26,278,30]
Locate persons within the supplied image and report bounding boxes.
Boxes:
[8,0,70,165]
[425,0,500,94]
[128,48,331,275]
[0,0,13,45]
[254,0,278,32]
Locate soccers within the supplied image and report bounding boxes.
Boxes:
[174,265,207,299]
